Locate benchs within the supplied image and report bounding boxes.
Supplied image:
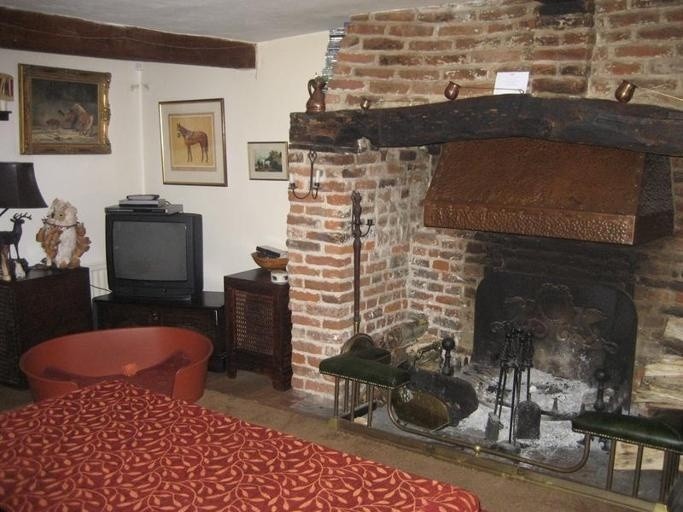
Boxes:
[2,383,478,512]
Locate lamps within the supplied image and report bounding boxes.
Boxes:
[0,161,49,276]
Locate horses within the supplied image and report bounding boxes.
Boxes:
[176,121,209,162]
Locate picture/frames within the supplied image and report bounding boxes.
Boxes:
[247,141,289,182]
[17,62,114,155]
[157,96,228,188]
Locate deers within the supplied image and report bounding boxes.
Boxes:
[0,212,33,260]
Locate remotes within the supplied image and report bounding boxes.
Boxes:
[127,195,158,200]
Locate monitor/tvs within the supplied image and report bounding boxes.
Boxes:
[105,212,204,302]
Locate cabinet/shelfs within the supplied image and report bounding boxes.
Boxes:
[224,269,292,389]
[93,292,226,376]
[0,267,94,391]
